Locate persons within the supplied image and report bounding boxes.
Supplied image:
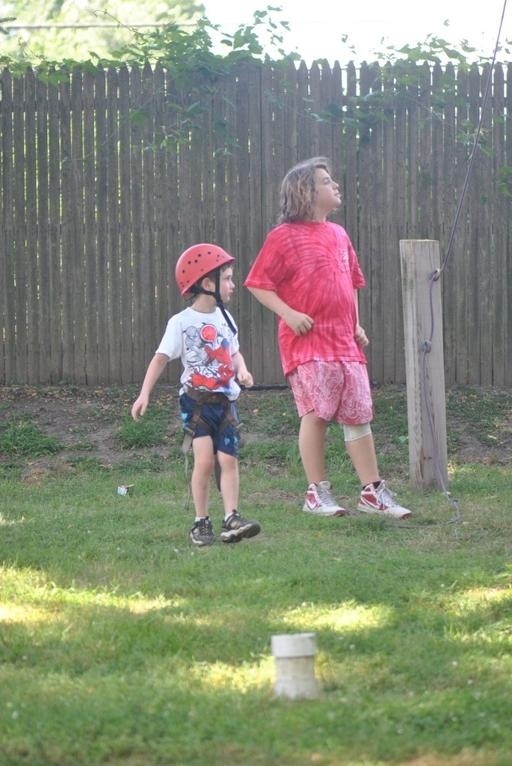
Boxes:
[129,241,262,547]
[242,155,414,520]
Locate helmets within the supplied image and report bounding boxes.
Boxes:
[175,243,235,297]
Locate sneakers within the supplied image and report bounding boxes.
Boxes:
[357,479,413,520]
[302,480,346,517]
[188,516,216,547]
[220,509,260,543]
